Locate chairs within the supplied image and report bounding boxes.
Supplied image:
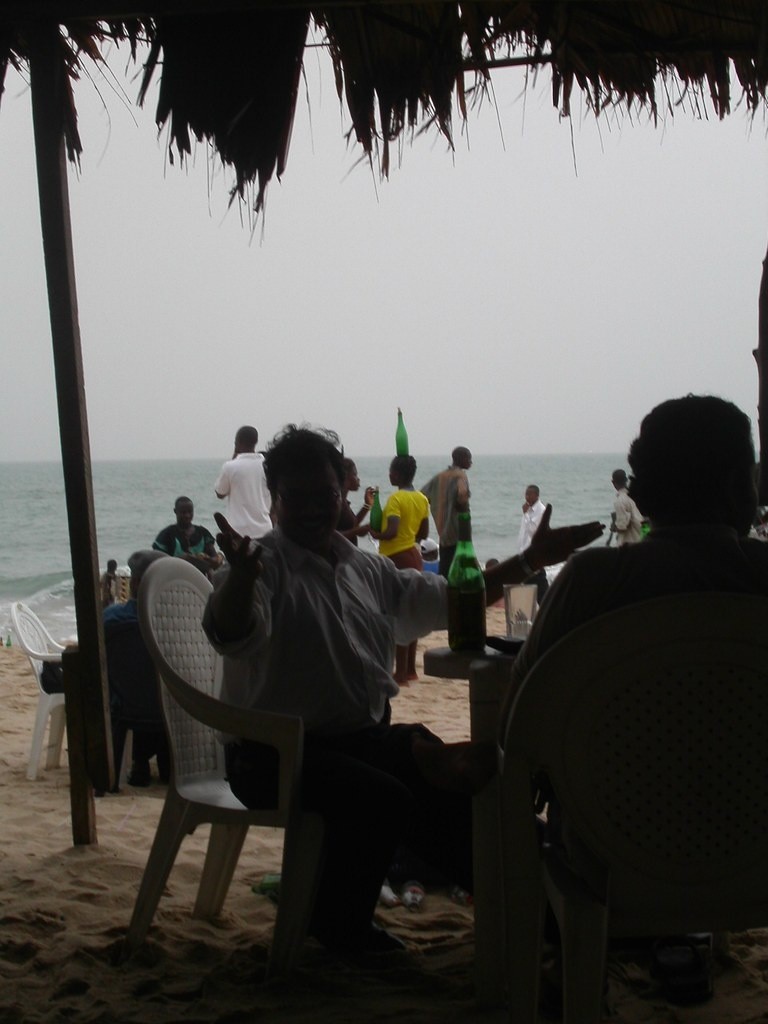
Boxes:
[491,591,768,1024]
[9,601,133,782]
[126,556,324,964]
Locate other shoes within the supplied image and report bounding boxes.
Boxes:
[647,935,713,1006]
[310,924,406,963]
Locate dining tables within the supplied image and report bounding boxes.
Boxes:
[424,641,522,1001]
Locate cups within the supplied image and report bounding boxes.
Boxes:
[501,583,538,641]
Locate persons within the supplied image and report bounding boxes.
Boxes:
[200,421,609,984]
[495,394,768,1008]
[151,495,224,578]
[79,550,180,789]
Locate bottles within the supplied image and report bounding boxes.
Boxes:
[370,486,384,536]
[396,410,408,457]
[6,635,12,647]
[443,501,488,654]
